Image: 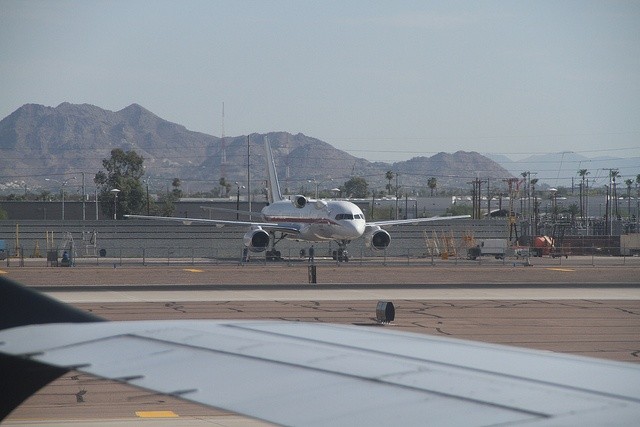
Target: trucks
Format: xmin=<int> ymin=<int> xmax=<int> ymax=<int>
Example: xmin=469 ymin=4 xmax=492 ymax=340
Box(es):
xmin=468 ymin=238 xmax=507 ymax=260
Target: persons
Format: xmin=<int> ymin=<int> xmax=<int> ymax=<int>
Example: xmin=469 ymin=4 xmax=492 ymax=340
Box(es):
xmin=62 ymin=251 xmax=70 ymax=263
xmin=535 ymin=233 xmax=546 ymax=257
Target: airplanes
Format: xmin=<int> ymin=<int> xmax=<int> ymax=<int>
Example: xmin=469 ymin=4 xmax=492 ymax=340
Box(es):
xmin=123 ymin=134 xmax=472 ymax=261
